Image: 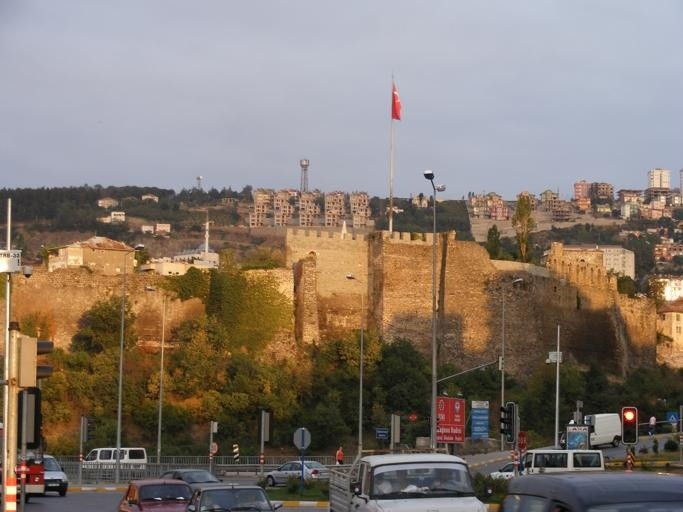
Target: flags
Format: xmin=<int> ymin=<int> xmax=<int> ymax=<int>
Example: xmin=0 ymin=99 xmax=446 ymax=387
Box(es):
xmin=390 ymin=80 xmax=402 ymax=123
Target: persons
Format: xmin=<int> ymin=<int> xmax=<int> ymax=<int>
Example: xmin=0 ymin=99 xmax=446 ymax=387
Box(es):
xmin=645 ymin=413 xmax=658 ymax=436
xmin=334 ymin=444 xmax=345 ymax=466
xmin=200 ymin=495 xmax=221 ymax=511
xmin=241 ymin=493 xmax=258 ymax=507
xmin=374 ymin=473 xmax=393 ymax=496
xmin=394 ymin=469 xmax=418 ymax=494
xmin=429 ymin=468 xmax=453 ymax=491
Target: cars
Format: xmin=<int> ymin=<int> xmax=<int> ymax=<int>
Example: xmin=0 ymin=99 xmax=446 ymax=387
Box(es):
xmin=40 ymin=455 xmax=70 ymax=496
xmin=264 ymin=459 xmax=330 ymax=487
xmin=118 ymin=466 xmax=285 ymax=511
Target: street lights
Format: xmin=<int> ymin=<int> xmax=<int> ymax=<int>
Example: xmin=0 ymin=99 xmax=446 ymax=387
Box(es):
xmin=146 ymin=282 xmax=162 ymax=463
xmin=423 ymin=167 xmax=443 ymax=450
xmin=345 ymin=272 xmax=365 ymax=450
xmin=497 ymin=278 xmax=523 ymax=446
xmin=115 ymin=242 xmax=145 ymax=482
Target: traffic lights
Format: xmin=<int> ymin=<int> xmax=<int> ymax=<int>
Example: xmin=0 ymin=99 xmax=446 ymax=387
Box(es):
xmin=498 ymin=405 xmax=515 ymax=435
xmin=19 ymin=336 xmax=54 ymax=387
xmin=623 ymin=411 xmax=635 ymax=442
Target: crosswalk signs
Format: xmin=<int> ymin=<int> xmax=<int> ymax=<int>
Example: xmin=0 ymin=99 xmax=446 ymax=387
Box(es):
xmin=667 ymin=411 xmax=679 ymax=422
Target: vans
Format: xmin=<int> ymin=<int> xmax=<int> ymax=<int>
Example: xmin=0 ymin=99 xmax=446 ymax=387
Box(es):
xmin=559 ymin=412 xmax=621 ymax=451
xmin=82 ymin=445 xmax=148 ymax=471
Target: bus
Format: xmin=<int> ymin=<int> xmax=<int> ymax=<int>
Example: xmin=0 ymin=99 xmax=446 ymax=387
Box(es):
xmin=0 ymin=410 xmax=47 ymax=506
